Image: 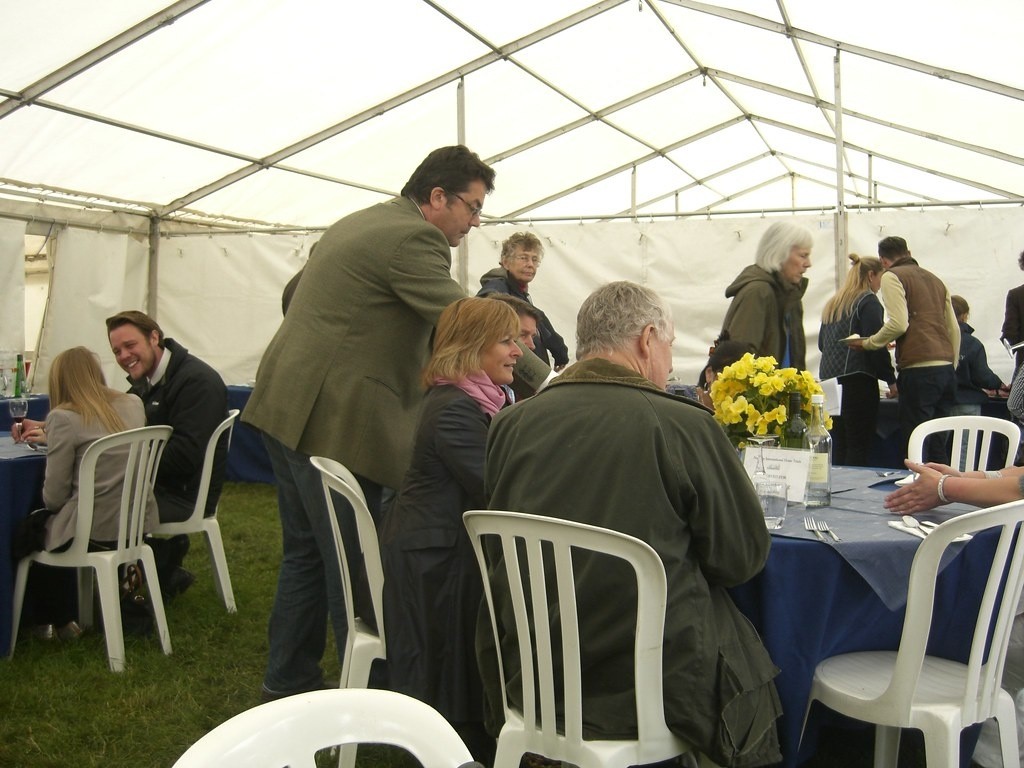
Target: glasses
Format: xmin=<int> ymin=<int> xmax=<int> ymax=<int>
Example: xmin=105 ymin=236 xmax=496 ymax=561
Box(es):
xmin=438 ymin=186 xmax=482 ymax=218
xmin=507 ymin=254 xmax=541 ymax=268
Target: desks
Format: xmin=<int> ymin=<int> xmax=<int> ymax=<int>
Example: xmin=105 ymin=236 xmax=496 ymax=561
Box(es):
xmin=0 ymin=431 xmax=47 ymax=656
xmin=749 ymin=463 xmax=1024 ymax=768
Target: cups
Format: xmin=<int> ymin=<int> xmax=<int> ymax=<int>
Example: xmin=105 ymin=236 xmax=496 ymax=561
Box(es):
xmin=755 ymin=483 xmax=787 ymax=530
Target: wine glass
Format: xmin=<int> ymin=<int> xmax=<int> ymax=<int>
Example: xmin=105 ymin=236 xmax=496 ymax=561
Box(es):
xmin=9 ymin=400 xmax=28 ymax=444
xmin=2 ymin=370 xmax=13 ymax=397
xmin=748 ymin=436 xmax=775 ymax=483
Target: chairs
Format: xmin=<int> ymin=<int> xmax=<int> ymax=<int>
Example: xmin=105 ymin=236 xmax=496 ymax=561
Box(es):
xmin=7 ymin=424 xmax=173 ymax=673
xmin=168 ymin=688 xmax=475 ymax=768
xmin=907 ymin=415 xmax=1020 ymax=477
xmin=463 ymin=511 xmax=691 ymax=768
xmin=310 ymin=455 xmax=385 ymax=768
xmin=792 ymin=500 xmax=1024 ymax=768
xmin=147 ymin=410 xmax=241 ymax=613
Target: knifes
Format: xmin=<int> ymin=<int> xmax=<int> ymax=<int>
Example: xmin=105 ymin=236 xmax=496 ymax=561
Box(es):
xmin=918 ymin=522 xmax=934 ymax=530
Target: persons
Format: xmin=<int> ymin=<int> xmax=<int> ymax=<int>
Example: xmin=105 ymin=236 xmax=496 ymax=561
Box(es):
xmin=238 ymin=145 xmax=559 ymax=698
xmin=11 ymin=311 xmax=229 ymax=644
xmin=488 ymin=281 xmax=784 ymax=768
xmin=883 ymin=459 xmax=1024 ymax=768
xmin=282 ymin=231 xmax=569 ymax=723
xmin=696 ymin=221 xmax=812 ymax=411
xmin=819 ymin=236 xmax=1024 ymax=471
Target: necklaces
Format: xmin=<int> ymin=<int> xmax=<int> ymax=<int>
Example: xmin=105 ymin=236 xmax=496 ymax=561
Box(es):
xmin=701 ymin=397 xmax=705 ymax=405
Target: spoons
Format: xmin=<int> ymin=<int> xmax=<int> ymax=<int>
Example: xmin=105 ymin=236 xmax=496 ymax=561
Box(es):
xmin=902 ymin=515 xmax=928 ymax=536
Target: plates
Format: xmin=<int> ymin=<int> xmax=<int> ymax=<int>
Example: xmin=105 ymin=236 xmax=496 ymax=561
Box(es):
xmin=839 ymin=337 xmax=868 ymax=343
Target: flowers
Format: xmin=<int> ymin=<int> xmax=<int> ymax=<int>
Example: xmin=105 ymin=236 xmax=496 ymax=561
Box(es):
xmin=709 ymin=352 xmax=834 ymax=447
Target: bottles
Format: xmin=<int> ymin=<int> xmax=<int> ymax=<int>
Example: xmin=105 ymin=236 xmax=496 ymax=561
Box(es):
xmin=803 ymin=394 xmax=832 ymax=506
xmin=779 ymin=393 xmax=807 ymax=450
xmin=15 ymin=354 xmax=27 ymax=398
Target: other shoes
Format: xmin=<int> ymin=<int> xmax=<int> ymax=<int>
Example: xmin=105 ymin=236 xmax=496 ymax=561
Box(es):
xmin=174 ymin=568 xmax=195 ymax=594
xmin=58 ymin=621 xmax=82 ymax=639
xmin=23 ymin=624 xmax=58 ymax=644
xmin=262 ymin=673 xmax=330 ymax=703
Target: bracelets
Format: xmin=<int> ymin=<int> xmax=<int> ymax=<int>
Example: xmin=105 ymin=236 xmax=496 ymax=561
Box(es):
xmin=983 ymin=471 xmax=1002 ymax=479
xmin=938 ymin=474 xmax=952 ymax=503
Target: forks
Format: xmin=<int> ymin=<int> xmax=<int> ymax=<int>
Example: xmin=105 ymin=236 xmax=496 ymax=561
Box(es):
xmin=804 ymin=517 xmax=824 ymax=541
xmin=817 ymin=521 xmax=840 ymax=541
xmin=876 ymin=472 xmax=894 ymax=476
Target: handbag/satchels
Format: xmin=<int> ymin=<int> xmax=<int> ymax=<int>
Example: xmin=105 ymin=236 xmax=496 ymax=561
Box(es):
xmin=119 ymin=564 xmax=153 ymax=635
